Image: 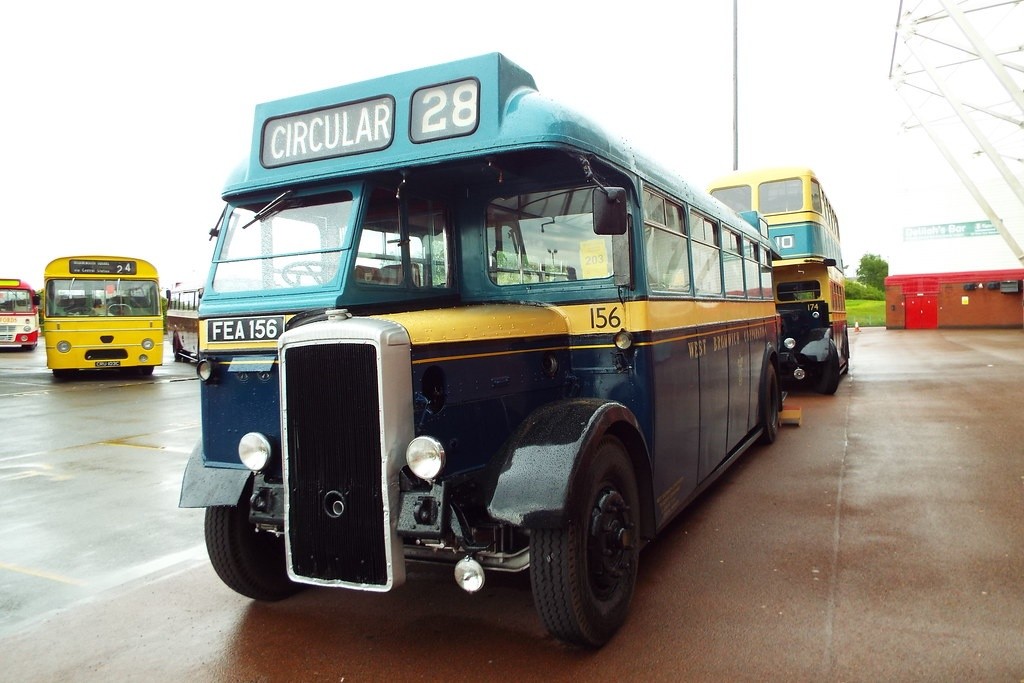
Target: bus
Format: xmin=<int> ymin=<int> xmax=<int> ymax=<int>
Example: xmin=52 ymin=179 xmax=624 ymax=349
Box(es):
xmin=32 ymin=255 xmax=163 ymax=376
xmin=704 ymin=164 xmax=849 ymax=396
xmin=166 ymin=287 xmax=203 ymax=363
xmin=0 ymin=279 xmax=39 ymax=350
xmin=178 ymin=52 xmax=780 ymax=654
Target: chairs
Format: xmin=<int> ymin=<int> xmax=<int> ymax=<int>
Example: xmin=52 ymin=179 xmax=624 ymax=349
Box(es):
xmin=374 ymin=262 xmax=421 ymax=288
